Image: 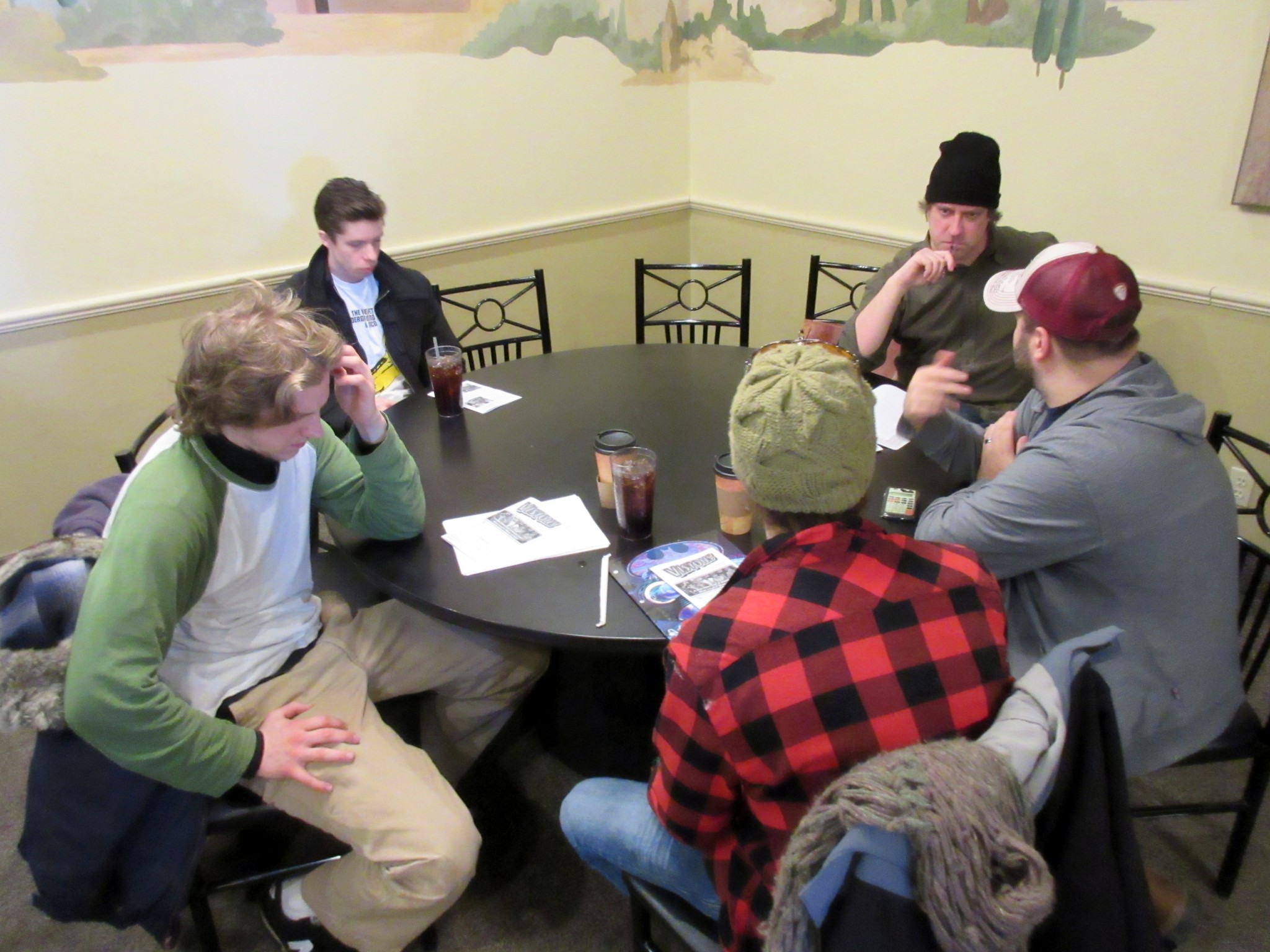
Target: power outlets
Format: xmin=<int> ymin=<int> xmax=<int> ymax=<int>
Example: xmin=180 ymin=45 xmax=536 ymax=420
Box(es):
xmin=1229 ymin=466 xmax=1255 ymax=508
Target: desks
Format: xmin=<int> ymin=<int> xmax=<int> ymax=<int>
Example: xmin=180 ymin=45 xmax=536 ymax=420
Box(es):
xmin=338 ymin=343 xmax=968 ymax=783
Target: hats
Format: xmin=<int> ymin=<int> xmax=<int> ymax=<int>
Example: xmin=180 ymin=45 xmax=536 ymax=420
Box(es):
xmin=924 ymin=131 xmax=1002 ymax=210
xmin=727 ymin=343 xmax=878 ymax=514
xmin=982 ymin=242 xmax=1143 ymax=344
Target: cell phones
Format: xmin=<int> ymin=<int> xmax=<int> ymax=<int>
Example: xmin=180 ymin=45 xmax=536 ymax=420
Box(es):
xmin=879 ymin=487 xmax=920 ymax=521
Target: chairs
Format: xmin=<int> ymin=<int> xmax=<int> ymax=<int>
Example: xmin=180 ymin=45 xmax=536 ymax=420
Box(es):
xmin=431 ymin=269 xmax=553 ymax=374
xmin=634 ymin=257 xmax=750 ymax=348
xmin=622 ymin=625 xmax=1177 ymax=952
xmin=1131 ymin=409 xmax=1270 ymax=899
xmin=804 ymin=254 xmax=882 ymax=325
xmin=0 ymin=469 xmax=422 ymax=952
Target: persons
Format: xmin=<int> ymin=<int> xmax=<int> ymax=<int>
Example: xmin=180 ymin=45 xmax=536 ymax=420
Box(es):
xmin=62 ymin=277 xmax=550 ymax=952
xmin=558 ymin=340 xmax=1016 ymax=952
xmin=896 ymin=236 xmax=1247 ymax=787
xmin=838 ymin=132 xmax=1059 ymax=429
xmin=271 ymin=176 xmax=467 ymax=440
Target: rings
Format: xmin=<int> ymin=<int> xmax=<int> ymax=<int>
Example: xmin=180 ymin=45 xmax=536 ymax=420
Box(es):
xmin=985 ymin=438 xmax=992 ymax=444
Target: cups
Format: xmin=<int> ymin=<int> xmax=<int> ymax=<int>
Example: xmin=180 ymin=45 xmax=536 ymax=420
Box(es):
xmin=425 ymin=346 xmax=464 ymax=418
xmin=610 ymin=447 xmax=657 ymax=541
xmin=713 ymin=452 xmax=753 ymax=535
xmin=594 ymin=429 xmax=637 ymax=483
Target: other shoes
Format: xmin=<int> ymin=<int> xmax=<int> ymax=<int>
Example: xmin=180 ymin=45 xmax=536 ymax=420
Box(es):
xmin=1144 ymin=865 xmax=1188 ymax=938
xmin=255 ymin=878 xmax=347 ymax=952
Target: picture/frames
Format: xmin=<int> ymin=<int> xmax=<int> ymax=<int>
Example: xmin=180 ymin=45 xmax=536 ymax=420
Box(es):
xmin=1231 ymin=33 xmax=1270 ymax=208
